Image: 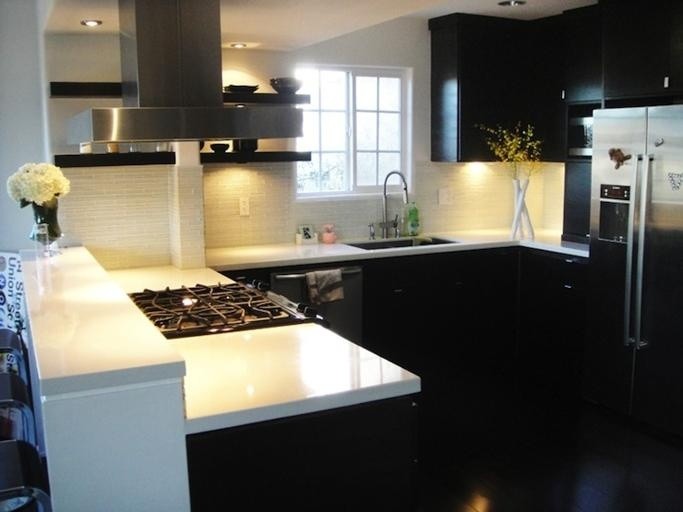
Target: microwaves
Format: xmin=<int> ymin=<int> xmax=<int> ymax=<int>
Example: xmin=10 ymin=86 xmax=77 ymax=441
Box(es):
xmin=566 ymin=114 xmax=601 ymax=157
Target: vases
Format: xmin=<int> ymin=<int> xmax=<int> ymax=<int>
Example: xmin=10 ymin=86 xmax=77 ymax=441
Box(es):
xmin=513 ymin=178 xmax=534 ymax=241
xmin=509 ymin=177 xmax=529 ymax=241
xmin=29 ymin=197 xmax=62 ymax=244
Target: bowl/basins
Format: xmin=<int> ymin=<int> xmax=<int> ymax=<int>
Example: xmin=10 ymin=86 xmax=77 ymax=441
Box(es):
xmin=198 ymin=139 xmax=204 ymax=152
xmin=210 ymin=144 xmax=229 ymax=153
xmin=232 ymin=138 xmax=258 ymax=151
xmin=269 ymin=73 xmax=301 ymax=94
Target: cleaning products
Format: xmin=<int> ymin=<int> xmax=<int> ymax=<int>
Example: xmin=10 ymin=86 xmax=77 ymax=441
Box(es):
xmin=405 ymin=202 xmax=422 ymax=236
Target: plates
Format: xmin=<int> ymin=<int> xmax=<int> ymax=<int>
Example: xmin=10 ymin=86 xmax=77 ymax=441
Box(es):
xmin=224 ymin=84 xmax=259 ymax=94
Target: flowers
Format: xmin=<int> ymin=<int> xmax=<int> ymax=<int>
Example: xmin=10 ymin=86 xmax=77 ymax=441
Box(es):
xmin=6 ymin=163 xmax=71 ymax=204
xmin=475 ymin=121 xmax=521 ymax=176
xmin=520 ymin=122 xmax=542 ymax=176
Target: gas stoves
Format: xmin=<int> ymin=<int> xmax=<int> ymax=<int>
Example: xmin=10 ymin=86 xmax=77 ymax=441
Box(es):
xmin=124 ymin=276 xmax=332 ymax=340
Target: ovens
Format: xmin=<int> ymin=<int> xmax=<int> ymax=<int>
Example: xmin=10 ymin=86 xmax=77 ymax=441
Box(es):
xmin=268 ymin=267 xmax=365 ymax=346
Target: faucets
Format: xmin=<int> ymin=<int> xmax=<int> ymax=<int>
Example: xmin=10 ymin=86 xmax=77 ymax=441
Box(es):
xmin=380 ymin=170 xmax=409 ymax=238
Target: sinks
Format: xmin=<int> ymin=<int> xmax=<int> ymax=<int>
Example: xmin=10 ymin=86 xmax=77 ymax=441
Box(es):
xmin=343 ymin=237 xmax=459 ymax=250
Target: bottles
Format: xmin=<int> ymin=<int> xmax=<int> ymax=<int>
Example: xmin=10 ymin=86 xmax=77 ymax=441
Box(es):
xmin=408 ymin=200 xmax=420 ymax=236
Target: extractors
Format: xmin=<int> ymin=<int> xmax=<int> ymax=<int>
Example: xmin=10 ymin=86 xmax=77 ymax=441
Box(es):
xmin=64 ymin=0 xmax=311 ymax=144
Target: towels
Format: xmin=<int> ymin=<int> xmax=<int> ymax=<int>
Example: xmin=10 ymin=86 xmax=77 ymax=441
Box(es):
xmin=306 ymin=268 xmax=344 ymax=305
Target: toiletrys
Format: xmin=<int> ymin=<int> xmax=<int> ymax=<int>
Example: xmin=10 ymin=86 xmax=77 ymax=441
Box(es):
xmin=322 ymin=224 xmax=339 ymax=244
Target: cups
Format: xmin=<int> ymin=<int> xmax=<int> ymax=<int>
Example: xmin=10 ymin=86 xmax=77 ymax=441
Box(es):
xmin=75 ymin=139 xmax=175 ymax=154
xmin=321 ymin=232 xmax=338 ymax=244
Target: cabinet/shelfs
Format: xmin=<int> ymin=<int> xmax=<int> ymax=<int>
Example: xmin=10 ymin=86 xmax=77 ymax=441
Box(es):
xmin=460 ymin=15 xmax=568 ymax=160
xmin=605 ymin=2 xmax=683 ymax=108
xmin=520 ymin=248 xmax=625 ymax=412
xmin=564 ymin=4 xmax=602 ymax=163
xmin=365 ymin=245 xmax=521 ymax=415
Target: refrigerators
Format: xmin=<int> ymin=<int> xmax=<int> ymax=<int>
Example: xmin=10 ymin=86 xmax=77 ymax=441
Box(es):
xmin=583 ymin=106 xmax=680 ymax=442
